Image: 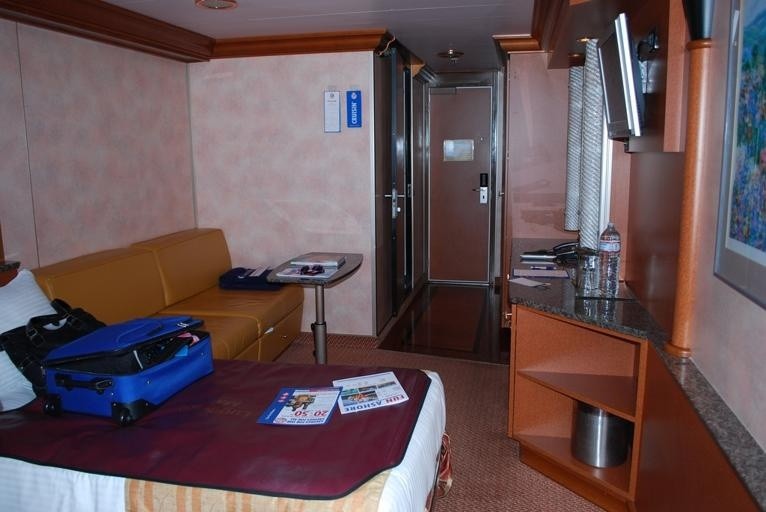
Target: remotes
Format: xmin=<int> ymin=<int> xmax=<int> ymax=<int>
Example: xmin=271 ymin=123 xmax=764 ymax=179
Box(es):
xmin=520 ymin=253 xmax=558 ymax=260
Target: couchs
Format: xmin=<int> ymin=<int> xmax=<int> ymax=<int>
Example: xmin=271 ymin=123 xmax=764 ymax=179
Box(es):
xmin=29 ymin=228 xmax=304 ymax=363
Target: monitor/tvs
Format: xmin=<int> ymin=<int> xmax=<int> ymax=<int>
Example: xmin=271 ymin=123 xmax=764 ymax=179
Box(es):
xmin=597 ymin=12 xmax=646 ymax=141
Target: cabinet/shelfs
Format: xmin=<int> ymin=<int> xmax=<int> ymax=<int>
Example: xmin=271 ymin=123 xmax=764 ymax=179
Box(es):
xmin=499 ymin=237 xmax=647 ymax=511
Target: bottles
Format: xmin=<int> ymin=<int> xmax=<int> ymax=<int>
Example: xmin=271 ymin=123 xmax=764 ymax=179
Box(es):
xmin=598 ymin=223 xmax=621 ymax=294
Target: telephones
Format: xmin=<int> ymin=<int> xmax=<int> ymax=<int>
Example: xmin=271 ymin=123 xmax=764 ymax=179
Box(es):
xmin=553 ymin=242 xmax=580 ymax=257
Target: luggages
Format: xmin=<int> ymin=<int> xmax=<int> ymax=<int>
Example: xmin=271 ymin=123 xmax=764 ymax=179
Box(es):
xmin=41 ymin=311 xmax=216 ymax=427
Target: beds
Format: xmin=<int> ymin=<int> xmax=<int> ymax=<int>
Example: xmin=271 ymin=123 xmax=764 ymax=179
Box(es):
xmin=0 ymin=266 xmax=452 ymax=512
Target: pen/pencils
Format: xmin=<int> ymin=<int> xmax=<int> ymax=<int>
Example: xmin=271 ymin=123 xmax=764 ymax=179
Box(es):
xmin=530 ymin=265 xmax=557 ymax=270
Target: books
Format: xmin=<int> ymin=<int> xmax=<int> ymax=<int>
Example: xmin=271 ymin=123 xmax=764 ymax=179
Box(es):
xmin=254 ymin=385 xmax=343 ymax=425
xmin=289 ymin=253 xmax=345 ymax=267
xmin=333 ymin=370 xmax=409 ymax=416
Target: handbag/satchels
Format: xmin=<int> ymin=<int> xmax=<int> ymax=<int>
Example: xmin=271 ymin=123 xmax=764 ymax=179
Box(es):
xmin=0 ymin=296 xmax=107 ymax=398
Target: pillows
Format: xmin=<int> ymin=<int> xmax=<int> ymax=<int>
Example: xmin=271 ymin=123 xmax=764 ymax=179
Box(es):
xmin=0 ymin=268 xmax=64 ymax=414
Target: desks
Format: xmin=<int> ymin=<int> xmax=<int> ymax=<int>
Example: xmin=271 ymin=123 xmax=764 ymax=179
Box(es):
xmin=266 ymin=251 xmax=363 ymax=366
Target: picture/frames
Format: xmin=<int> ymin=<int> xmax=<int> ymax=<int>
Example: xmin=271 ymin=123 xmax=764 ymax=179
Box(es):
xmin=711 ymin=1 xmax=766 ymax=314
